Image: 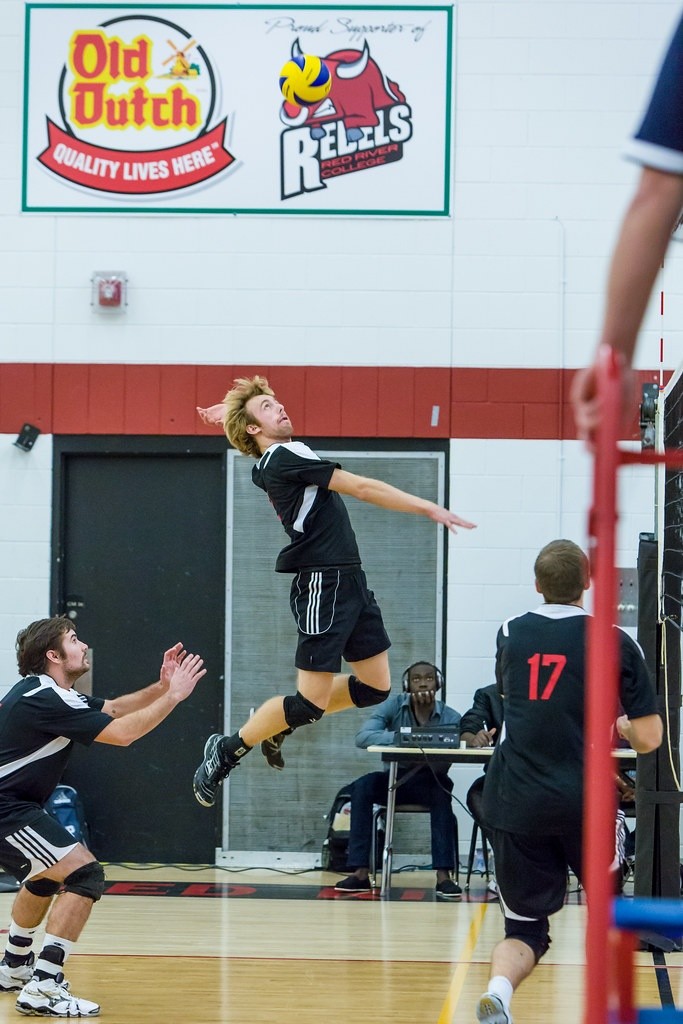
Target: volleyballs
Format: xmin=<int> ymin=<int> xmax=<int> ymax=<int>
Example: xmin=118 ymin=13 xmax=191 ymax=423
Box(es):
xmin=280 ymin=54 xmax=332 ymax=107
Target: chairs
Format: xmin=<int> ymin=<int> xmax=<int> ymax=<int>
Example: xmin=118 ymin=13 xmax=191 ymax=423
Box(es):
xmin=371 ymin=805 xmax=460 ymax=888
xmin=465 ymin=820 xmax=491 ymax=892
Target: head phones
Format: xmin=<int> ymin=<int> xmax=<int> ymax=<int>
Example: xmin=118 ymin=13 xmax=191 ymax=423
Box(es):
xmin=402 ymin=664 xmax=445 ymax=694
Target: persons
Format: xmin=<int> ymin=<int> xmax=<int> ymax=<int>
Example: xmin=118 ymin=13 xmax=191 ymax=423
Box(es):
xmin=570 ymin=0 xmax=683 ymax=454
xmin=334 ymin=660 xmax=462 ymax=896
xmin=193 ymin=376 xmax=477 ymax=807
xmin=0 ymin=617 xmax=207 ymax=1018
xmin=479 ymin=540 xmax=663 ymax=1024
xmin=459 ymin=683 xmax=504 ymax=829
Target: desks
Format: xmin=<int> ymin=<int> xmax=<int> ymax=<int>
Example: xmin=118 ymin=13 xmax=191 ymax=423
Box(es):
xmin=366 ymin=746 xmax=638 ymax=898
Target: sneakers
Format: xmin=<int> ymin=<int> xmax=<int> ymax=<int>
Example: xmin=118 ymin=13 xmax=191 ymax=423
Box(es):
xmin=249 ymin=708 xmax=287 ymax=771
xmin=190 ymin=730 xmax=242 ymax=810
xmin=488 ymin=877 xmax=499 ymax=897
xmin=476 ymin=991 xmax=512 ymax=1024
xmin=13 ymin=980 xmax=102 ymax=1017
xmin=0 ymin=957 xmax=38 ymax=991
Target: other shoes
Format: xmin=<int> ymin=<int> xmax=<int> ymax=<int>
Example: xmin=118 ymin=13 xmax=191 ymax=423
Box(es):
xmin=334 ymin=874 xmax=372 ymax=894
xmin=435 ymin=877 xmax=463 ymax=898
